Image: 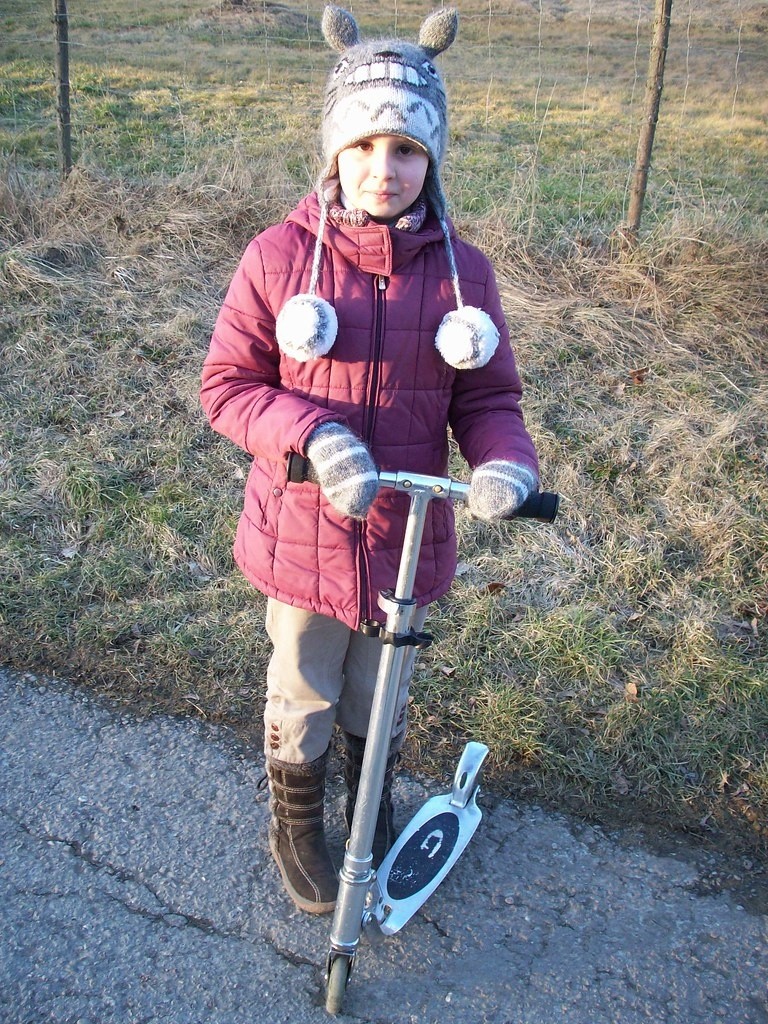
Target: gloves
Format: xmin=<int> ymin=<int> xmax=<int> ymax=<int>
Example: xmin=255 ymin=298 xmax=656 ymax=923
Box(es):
xmin=304 ymin=420 xmax=378 ymax=520
xmin=463 ymin=458 xmax=539 ymax=522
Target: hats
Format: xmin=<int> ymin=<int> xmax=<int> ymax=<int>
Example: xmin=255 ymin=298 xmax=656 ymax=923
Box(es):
xmin=316 ymin=5 xmax=458 ymax=223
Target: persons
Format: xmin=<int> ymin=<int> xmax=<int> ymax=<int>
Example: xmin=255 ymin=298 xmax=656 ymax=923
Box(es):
xmin=198 ymin=5 xmax=540 ymax=913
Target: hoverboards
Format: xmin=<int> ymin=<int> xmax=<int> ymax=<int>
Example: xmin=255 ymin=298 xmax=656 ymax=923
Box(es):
xmin=283 ymin=447 xmax=560 ymax=1012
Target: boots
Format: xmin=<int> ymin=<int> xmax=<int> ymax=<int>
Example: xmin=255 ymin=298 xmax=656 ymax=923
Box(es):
xmin=340 ymin=729 xmax=406 ymax=873
xmin=262 ymin=754 xmax=340 ymax=913
